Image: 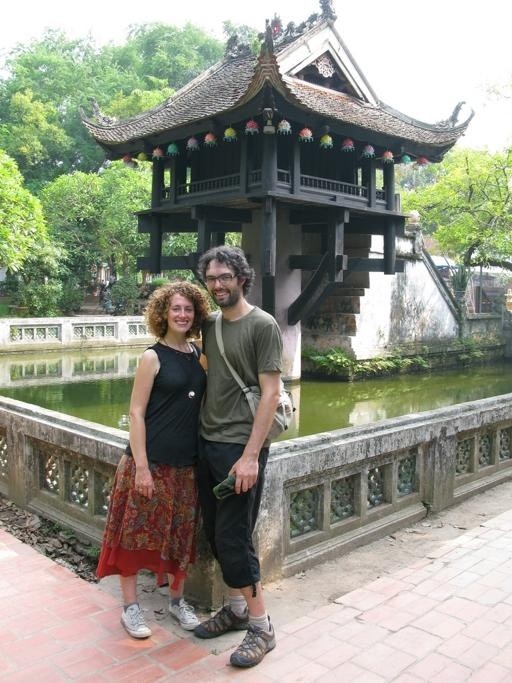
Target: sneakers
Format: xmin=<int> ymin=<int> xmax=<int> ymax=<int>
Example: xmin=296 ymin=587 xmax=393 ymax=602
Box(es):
xmin=194 ymin=604 xmax=248 ymax=643
xmin=121 ymin=603 xmax=152 ymax=639
xmin=230 ymin=615 xmax=275 ymax=667
xmin=168 ymin=598 xmax=202 ymax=630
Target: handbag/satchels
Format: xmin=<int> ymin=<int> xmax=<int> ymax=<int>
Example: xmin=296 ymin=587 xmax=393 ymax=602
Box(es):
xmin=245 ymin=380 xmax=294 ymax=439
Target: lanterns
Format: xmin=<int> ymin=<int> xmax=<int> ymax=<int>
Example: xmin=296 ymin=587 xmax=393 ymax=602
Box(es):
xmin=122 ymin=118 xmax=259 ymax=168
xmin=279 ymin=118 xmax=430 ymax=170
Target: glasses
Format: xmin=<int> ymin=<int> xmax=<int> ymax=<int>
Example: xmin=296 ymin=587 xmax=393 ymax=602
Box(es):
xmin=204 ymin=273 xmax=239 ymax=285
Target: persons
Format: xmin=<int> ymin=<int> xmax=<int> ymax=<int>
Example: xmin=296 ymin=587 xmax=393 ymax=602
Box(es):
xmin=93 ymin=282 xmax=210 ymax=640
xmin=190 ymin=245 xmax=284 ymax=669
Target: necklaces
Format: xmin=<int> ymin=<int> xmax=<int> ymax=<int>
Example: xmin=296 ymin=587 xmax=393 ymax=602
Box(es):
xmin=163 ymin=338 xmax=191 ymax=353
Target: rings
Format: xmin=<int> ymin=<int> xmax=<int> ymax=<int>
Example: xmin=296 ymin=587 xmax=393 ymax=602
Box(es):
xmin=138 ymin=489 xmax=143 ymax=492
